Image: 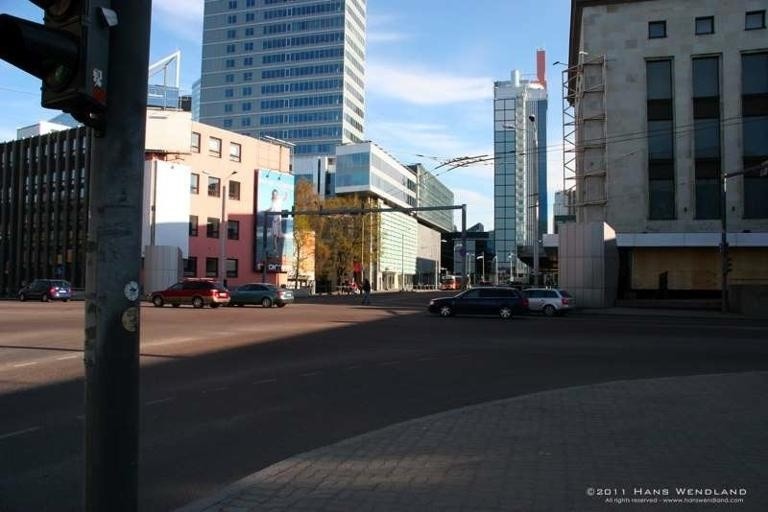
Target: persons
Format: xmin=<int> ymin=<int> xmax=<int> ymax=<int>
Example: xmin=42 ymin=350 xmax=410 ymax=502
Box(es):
xmin=363 ymin=279 xmax=374 ymax=309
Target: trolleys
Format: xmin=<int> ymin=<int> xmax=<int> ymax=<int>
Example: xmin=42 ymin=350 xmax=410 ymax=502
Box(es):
xmin=349 ymin=288 xmax=364 ymax=304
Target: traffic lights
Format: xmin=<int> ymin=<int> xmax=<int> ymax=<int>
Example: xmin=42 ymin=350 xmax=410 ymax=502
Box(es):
xmin=0 ymin=0 xmax=119 ymax=138
xmin=721 ymin=254 xmax=734 ymax=276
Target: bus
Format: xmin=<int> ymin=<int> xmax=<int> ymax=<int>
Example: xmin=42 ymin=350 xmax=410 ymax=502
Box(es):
xmin=440 ymin=274 xmax=465 ymax=290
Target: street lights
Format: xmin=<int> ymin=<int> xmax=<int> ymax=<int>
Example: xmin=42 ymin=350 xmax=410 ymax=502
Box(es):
xmin=453 ymin=243 xmax=519 ymax=288
xmin=402 ymin=227 xmax=414 ymax=293
xmin=435 ymin=239 xmax=448 ymax=290
xmin=498 ymin=114 xmax=539 ymax=291
xmin=218 ymin=170 xmax=240 ymax=285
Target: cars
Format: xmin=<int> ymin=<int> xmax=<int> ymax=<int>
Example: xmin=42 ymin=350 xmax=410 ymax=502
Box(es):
xmin=223 ymin=283 xmax=297 ymax=310
xmin=150 ymin=276 xmax=234 ymax=311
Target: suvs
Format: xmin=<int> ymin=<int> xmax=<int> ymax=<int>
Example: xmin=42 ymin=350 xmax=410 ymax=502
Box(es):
xmin=426 ymin=285 xmax=527 ymax=320
xmin=518 ymin=287 xmax=577 ymax=317
xmin=16 ymin=277 xmax=73 ymax=303
